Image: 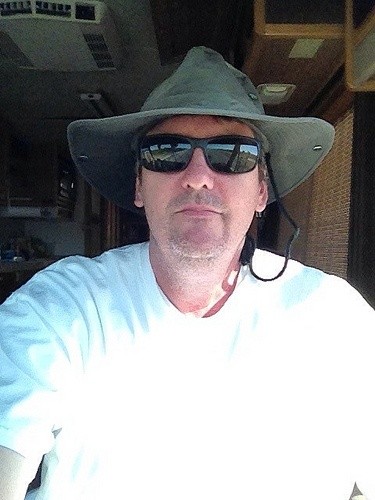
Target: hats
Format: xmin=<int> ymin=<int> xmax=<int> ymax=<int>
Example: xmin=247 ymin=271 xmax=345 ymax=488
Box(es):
xmin=67 ymin=46 xmax=337 ymax=216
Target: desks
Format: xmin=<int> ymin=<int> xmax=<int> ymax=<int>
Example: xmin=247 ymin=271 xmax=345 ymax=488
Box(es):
xmin=0 ymin=255 xmax=58 ymax=282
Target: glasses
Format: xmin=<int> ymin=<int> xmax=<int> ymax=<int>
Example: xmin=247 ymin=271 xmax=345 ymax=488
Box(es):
xmin=135 ymin=133 xmax=266 ymax=175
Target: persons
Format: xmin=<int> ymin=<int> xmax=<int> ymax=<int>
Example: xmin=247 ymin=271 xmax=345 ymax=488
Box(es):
xmin=0 ymin=46 xmax=375 ymax=500
